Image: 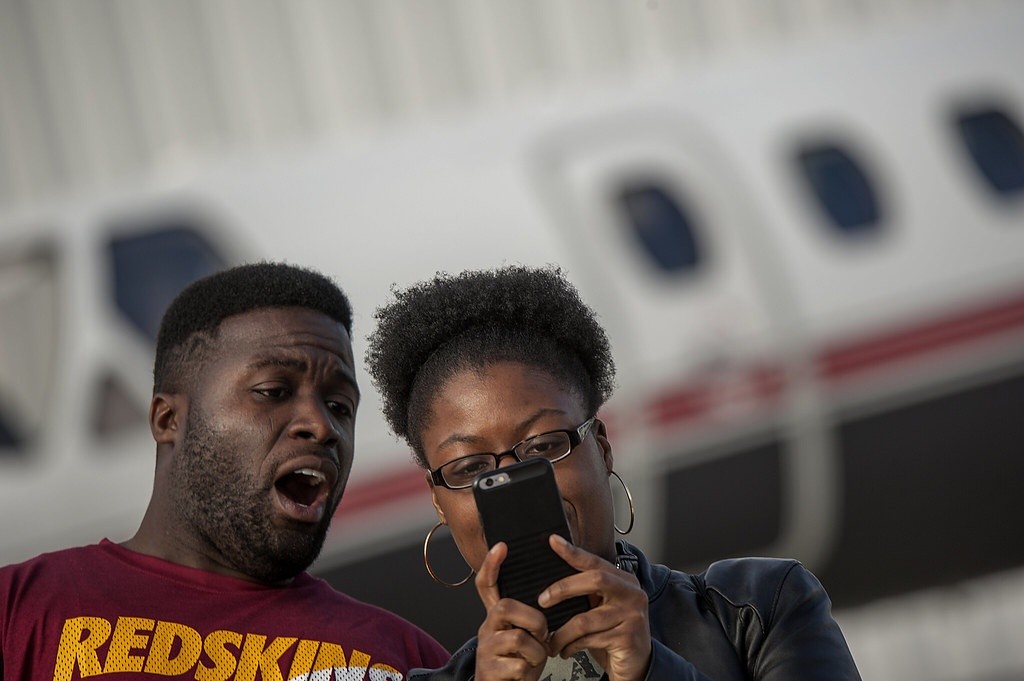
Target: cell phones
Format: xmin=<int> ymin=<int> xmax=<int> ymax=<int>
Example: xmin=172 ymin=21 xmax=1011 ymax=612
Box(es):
xmin=473 ymin=457 xmax=591 ymax=636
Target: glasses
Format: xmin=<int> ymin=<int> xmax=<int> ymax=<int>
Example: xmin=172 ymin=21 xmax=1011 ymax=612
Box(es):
xmin=423 ymin=415 xmax=597 ymax=490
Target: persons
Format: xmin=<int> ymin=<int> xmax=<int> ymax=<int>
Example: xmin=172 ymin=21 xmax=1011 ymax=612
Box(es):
xmin=370 ymin=267 xmax=864 ymax=681
xmin=0 ymin=262 xmax=452 ymax=681
